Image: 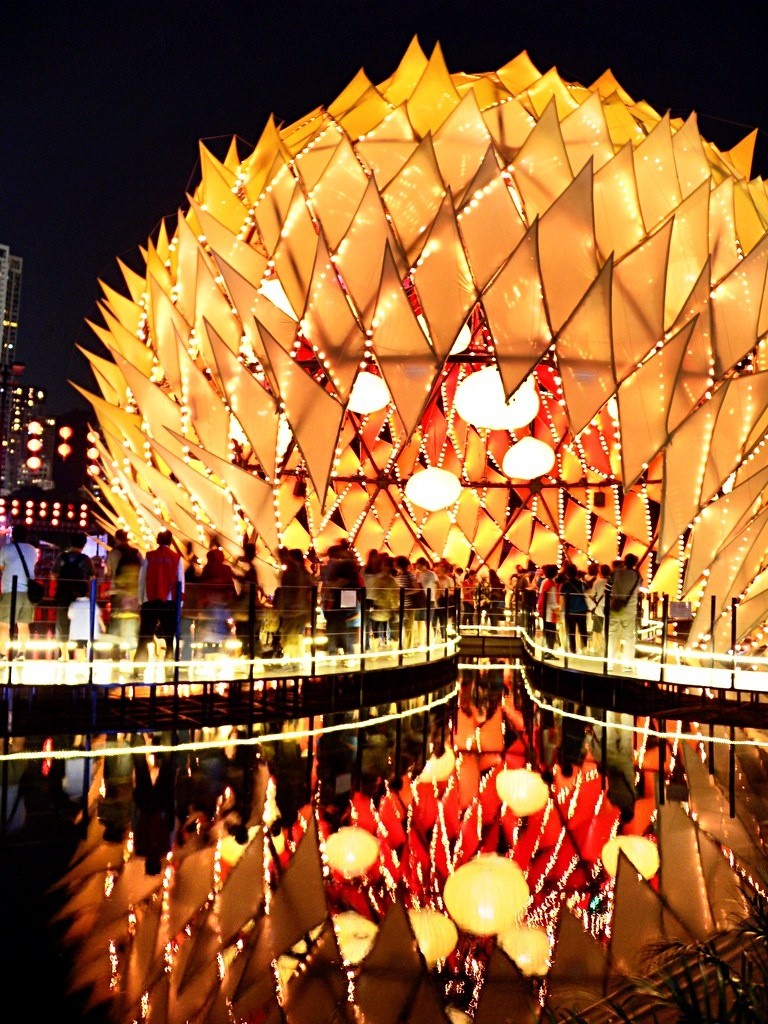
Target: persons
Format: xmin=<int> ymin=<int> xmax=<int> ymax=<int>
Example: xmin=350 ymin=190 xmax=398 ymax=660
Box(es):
xmin=17 ymin=730 xmax=323 ymax=876
xmin=520 ymin=688 xmax=689 ymax=820
xmin=0 ymin=524 xmax=625 ymax=660
xmin=317 ymin=691 xmax=463 ymax=826
xmin=462 ymin=657 xmax=511 ymax=727
xmin=603 ymin=553 xmax=643 ymax=671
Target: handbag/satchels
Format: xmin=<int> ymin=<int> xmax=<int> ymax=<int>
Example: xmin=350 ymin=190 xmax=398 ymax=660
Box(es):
xmin=610 ymin=596 xmax=625 ymax=612
xmin=592 ymin=614 xmax=604 ymax=632
xmin=407 ymin=571 xmax=428 ymax=609
xmin=27 ymin=578 xmax=46 ymax=604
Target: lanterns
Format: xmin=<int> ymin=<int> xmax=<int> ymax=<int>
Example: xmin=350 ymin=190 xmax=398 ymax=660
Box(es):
xmin=0 ymin=419 xmax=99 ymax=537
